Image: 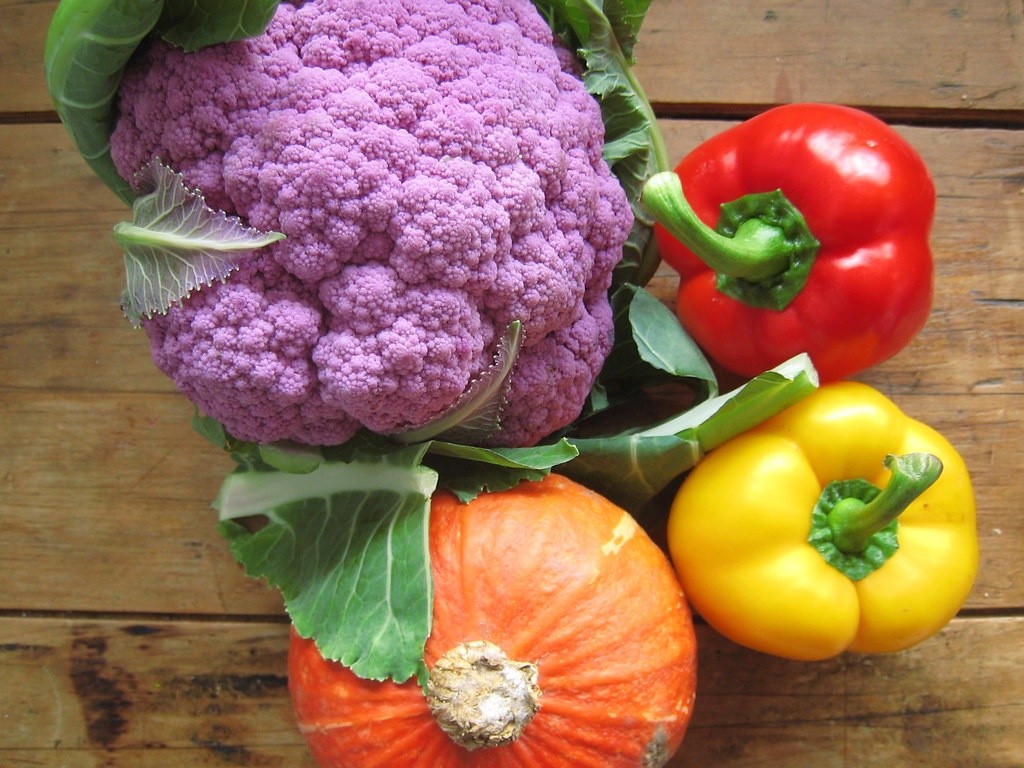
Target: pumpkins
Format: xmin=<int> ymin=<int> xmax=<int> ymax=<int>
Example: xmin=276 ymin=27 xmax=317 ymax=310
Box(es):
xmin=286 ymin=475 xmax=698 ymax=767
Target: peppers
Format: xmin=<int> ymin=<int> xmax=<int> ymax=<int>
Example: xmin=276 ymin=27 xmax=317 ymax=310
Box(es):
xmin=665 ymin=381 xmax=978 ymax=661
xmin=639 ymin=100 xmax=935 ymax=387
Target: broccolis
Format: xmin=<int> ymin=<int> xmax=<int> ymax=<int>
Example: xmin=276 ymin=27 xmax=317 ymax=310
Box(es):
xmin=44 ymin=0 xmax=820 ymax=682
